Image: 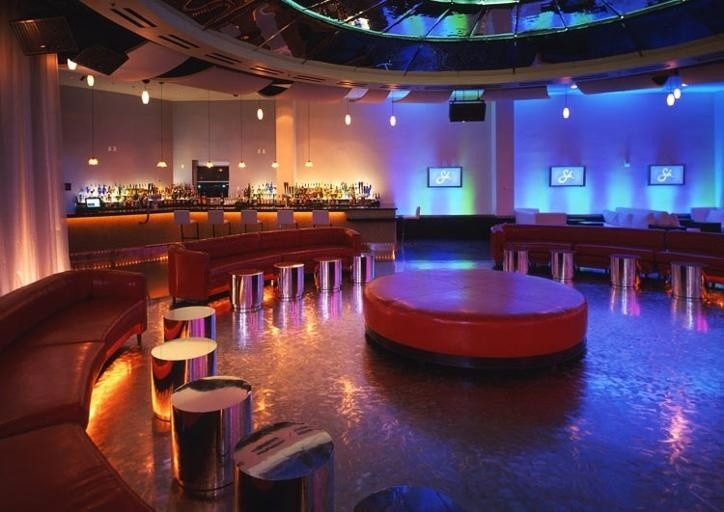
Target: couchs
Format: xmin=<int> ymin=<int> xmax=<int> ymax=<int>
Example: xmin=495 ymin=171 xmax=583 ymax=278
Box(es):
xmin=0 ymin=422 xmax=155 ymax=512
xmin=1 ymin=269 xmax=146 ymax=438
xmin=491 ymin=202 xmax=724 ymax=287
xmin=169 ymin=226 xmax=361 ymax=307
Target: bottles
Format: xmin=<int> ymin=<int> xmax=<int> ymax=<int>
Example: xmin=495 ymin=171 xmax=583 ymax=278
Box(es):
xmin=234 ymin=180 xmax=375 ymax=207
xmin=78 ymin=183 xmax=207 ymax=210
xmin=220 ymin=192 xmax=225 ymax=205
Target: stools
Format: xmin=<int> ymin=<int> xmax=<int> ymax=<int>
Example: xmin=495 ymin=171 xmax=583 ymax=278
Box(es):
xmin=503 ymin=247 xmax=702 ymax=300
xmin=363 ymin=270 xmax=588 ymax=378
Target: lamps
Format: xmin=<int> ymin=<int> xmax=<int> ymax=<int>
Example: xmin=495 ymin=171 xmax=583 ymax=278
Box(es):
xmin=86 ymin=75 xmax=94 ymax=87
xmin=89 ymin=90 xmax=98 ymax=165
xmin=345 ymin=100 xmax=351 ymax=126
xmin=257 ymin=94 xmax=264 ymax=120
xmin=563 ymin=85 xmax=570 ymax=119
xmin=667 ymin=77 xmax=675 ymax=107
xmin=157 ymin=81 xmax=168 ymax=169
xmin=272 ymin=99 xmax=279 ymax=168
xmin=205 ymin=89 xmax=213 ymax=168
xmin=674 ymin=75 xmax=681 ymax=100
xmin=390 ymin=102 xmax=396 ymax=126
xmin=304 ymin=101 xmax=313 ymax=166
xmin=141 ymin=79 xmax=150 ymax=104
xmin=238 ymin=95 xmax=246 ymax=169
xmin=67 ymin=59 xmax=78 ymax=72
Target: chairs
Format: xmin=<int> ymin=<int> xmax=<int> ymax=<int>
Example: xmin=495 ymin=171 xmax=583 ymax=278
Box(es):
xmin=312 ymin=210 xmax=332 ymax=228
xmin=277 ymin=210 xmax=298 ymax=229
xmin=208 ymin=209 xmax=231 ymax=237
xmin=400 ymin=206 xmax=420 ymax=247
xmin=241 ymin=210 xmax=263 ymax=232
xmin=174 ymin=210 xmax=198 ymax=241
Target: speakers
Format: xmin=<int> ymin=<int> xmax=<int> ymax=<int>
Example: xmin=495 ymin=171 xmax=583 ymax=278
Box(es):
xmin=8 ymin=14 xmax=80 ymax=56
xmin=70 ymin=39 xmax=129 ymax=76
xmin=449 ymin=99 xmax=487 ymax=123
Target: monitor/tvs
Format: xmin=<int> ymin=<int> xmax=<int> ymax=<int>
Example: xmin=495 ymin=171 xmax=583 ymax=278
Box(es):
xmin=549 ymin=166 xmax=586 ymax=187
xmin=647 ymin=163 xmax=685 ymax=186
xmin=427 ymin=166 xmax=462 ymax=188
